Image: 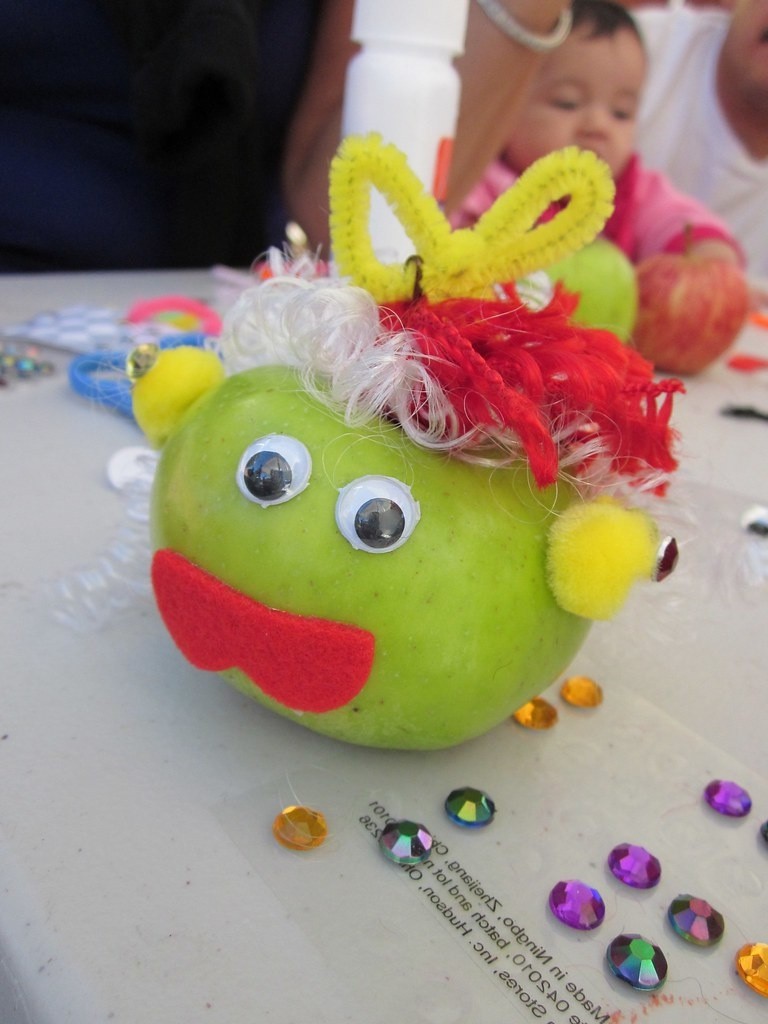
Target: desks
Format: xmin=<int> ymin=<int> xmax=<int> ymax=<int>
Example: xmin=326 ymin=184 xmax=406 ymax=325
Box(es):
xmin=0 ymin=269 xmax=768 ymax=1024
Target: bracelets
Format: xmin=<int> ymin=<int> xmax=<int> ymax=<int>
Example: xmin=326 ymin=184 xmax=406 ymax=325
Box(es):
xmin=478 ymin=0 xmax=576 ymax=53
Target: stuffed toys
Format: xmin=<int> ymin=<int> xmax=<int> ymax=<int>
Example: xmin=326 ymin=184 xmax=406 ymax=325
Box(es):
xmin=120 ymin=134 xmax=692 ymax=753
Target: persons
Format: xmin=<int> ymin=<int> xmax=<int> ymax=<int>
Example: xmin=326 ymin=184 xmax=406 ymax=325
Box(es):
xmin=441 ymin=2 xmax=766 ymax=286
xmin=448 ymin=6 xmax=753 ymax=284
xmin=0 ymin=0 xmax=358 ymax=270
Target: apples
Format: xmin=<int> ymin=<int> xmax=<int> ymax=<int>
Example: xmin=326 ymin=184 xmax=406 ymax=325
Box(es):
xmin=534 ymin=222 xmax=751 ymax=377
xmin=150 ymin=359 xmax=594 ymax=750
